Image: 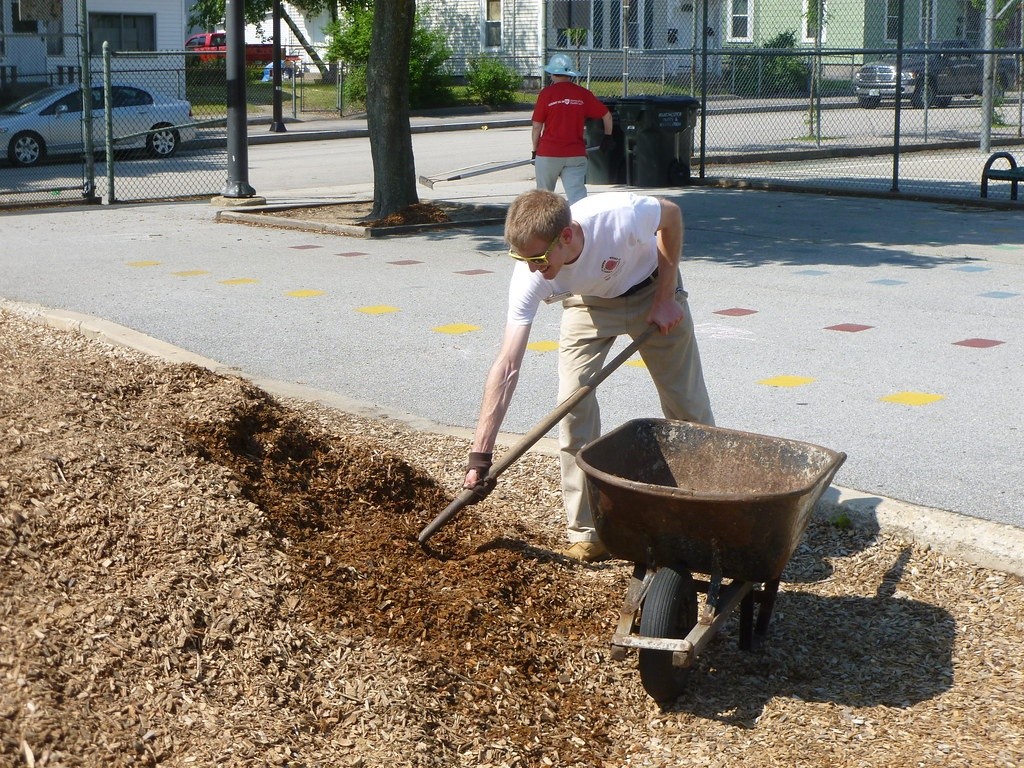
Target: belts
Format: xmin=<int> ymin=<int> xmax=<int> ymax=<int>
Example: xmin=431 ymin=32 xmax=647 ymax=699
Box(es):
xmin=617 ymin=266 xmax=659 ymax=298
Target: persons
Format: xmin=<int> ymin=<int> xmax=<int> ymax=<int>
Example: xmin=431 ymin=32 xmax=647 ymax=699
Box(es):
xmin=532 ymin=54 xmax=612 ymax=205
xmin=463 ymin=190 xmax=716 ymax=561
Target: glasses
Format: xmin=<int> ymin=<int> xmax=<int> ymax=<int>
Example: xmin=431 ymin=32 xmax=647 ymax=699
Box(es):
xmin=508 ymin=234 xmax=561 ymax=266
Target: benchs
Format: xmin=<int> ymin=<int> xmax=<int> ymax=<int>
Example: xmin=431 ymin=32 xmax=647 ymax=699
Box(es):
xmin=980 ymin=152 xmax=1024 ymax=200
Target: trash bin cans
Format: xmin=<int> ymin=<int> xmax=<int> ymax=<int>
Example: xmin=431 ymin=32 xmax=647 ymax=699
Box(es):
xmin=584 ymin=96 xmax=627 ymax=185
xmin=615 ymin=94 xmax=702 ymax=188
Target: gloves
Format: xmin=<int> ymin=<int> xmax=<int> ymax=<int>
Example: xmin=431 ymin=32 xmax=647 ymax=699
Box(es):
xmin=531 ymin=151 xmax=536 ymax=165
xmin=599 ymin=135 xmax=616 ymax=152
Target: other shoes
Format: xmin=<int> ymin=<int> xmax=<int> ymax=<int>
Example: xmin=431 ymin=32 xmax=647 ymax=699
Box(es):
xmin=566 ymin=541 xmax=609 ymax=563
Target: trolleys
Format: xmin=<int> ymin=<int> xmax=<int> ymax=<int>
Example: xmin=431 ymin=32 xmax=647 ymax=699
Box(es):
xmin=576 ymin=419 xmax=849 ymax=701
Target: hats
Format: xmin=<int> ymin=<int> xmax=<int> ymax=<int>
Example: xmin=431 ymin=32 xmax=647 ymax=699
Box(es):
xmin=544 ymin=54 xmax=581 ymax=76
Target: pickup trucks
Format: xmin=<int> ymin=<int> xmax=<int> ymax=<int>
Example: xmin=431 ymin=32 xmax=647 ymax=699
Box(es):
xmin=186 ymin=34 xmax=274 ymax=71
xmin=852 ymin=38 xmax=1019 ymax=109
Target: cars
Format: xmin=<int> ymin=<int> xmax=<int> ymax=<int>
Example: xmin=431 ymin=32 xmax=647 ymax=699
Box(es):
xmin=0 ymin=82 xmax=195 ymax=169
